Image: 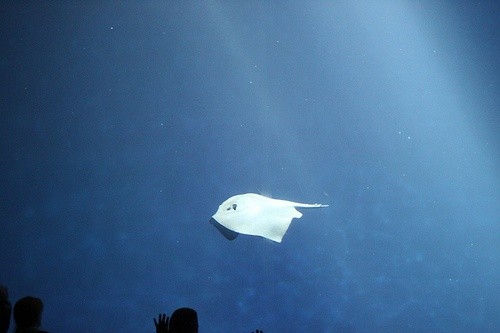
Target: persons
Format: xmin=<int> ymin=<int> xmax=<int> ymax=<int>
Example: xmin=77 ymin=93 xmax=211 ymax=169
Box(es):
xmin=153 ymin=307 xmax=267 ymax=333
xmin=0 ymin=284 xmax=49 ymax=333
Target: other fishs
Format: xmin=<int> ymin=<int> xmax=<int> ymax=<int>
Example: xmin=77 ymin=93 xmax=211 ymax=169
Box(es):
xmin=209 ymin=193 xmax=330 ymax=243
